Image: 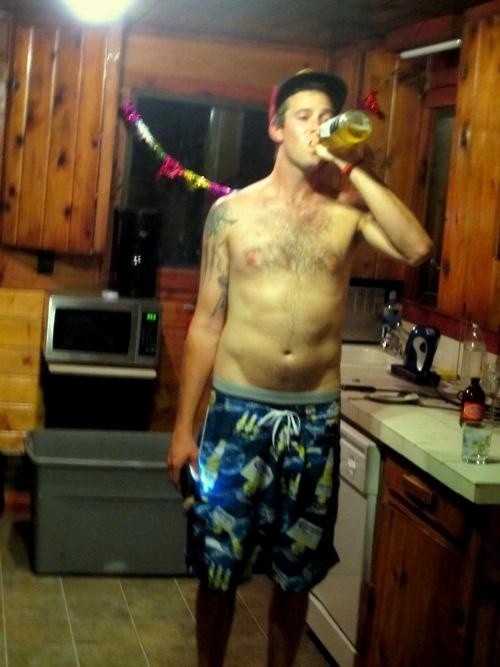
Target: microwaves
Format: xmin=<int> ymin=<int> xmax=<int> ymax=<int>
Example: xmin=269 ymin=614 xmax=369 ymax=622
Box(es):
xmin=41 ymin=288 xmax=162 ymax=367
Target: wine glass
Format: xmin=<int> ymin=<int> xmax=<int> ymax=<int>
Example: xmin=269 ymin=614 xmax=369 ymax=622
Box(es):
xmin=480 ymin=365 xmax=500 ymax=433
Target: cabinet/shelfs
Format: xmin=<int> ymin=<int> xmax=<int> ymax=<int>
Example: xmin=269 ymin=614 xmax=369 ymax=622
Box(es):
xmin=0 ymin=8 xmax=127 ymax=261
xmin=356 ymin=450 xmax=500 ymax=667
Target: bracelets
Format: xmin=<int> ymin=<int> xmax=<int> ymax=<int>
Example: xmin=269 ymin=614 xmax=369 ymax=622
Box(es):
xmin=343 ymin=156 xmax=361 ymax=177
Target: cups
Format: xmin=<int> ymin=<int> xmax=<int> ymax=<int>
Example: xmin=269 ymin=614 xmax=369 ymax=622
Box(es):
xmin=462 ymin=420 xmax=491 ymax=464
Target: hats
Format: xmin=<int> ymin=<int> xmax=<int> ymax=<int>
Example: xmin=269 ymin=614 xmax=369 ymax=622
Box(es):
xmin=272 ymin=67 xmax=346 ymax=117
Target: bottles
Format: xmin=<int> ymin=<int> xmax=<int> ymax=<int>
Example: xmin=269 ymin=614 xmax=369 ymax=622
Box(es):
xmin=383 ymin=291 xmax=403 ymax=354
xmin=318 ymin=111 xmax=372 ymax=149
xmin=456 ymin=320 xmax=485 ymax=428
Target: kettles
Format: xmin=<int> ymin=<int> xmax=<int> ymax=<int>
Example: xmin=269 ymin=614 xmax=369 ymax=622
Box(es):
xmin=405 ymin=325 xmax=441 ymax=376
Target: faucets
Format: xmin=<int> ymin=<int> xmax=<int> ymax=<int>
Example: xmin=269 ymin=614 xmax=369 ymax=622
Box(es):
xmin=381 ymin=331 xmax=405 ymax=358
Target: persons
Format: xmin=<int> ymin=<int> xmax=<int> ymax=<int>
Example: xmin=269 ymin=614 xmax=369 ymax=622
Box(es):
xmin=163 ymin=66 xmax=437 ymax=665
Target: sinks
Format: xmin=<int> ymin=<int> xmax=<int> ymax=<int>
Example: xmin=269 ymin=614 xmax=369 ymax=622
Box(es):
xmin=340 ymin=344 xmax=405 ymax=367
xmin=338 ymin=363 xmax=405 ymax=395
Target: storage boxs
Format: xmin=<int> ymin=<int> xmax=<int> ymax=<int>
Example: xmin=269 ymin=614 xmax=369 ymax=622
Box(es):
xmin=22 ymin=427 xmax=196 ymax=576
